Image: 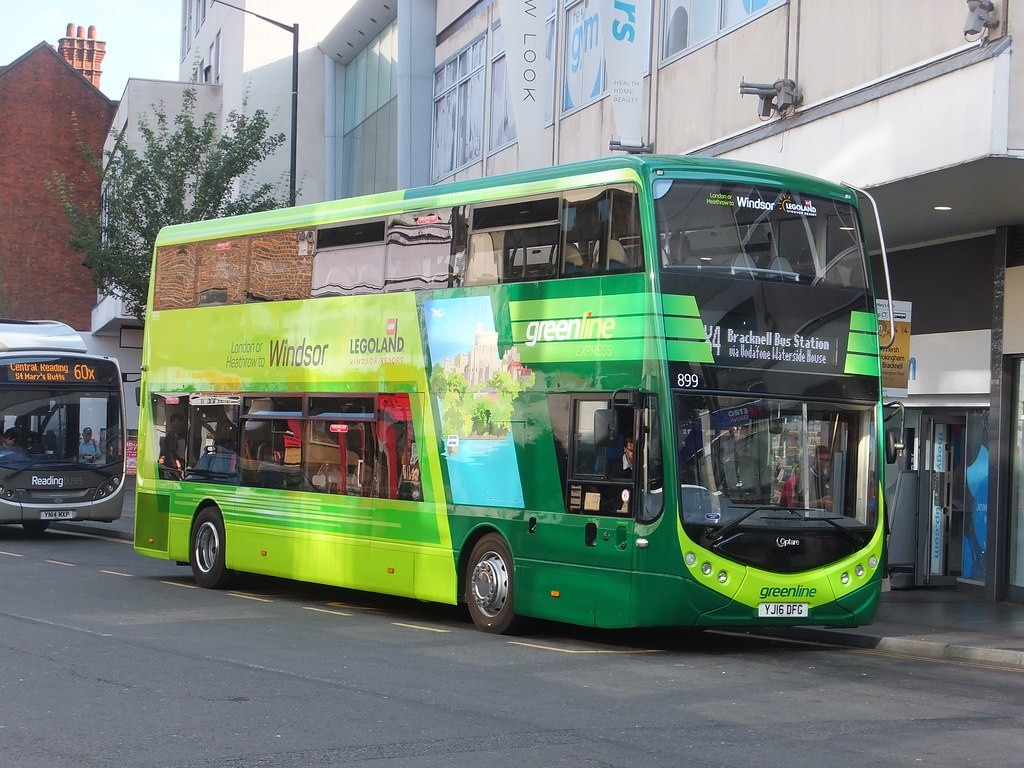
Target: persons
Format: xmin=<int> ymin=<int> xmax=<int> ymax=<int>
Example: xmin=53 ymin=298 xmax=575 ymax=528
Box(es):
xmin=607 ymin=436 xmax=633 ymax=478
xmin=157 ymin=411 xmax=189 ymax=478
xmin=0 ymin=414 xmax=29 ymax=447
xmin=79 ymin=427 xmax=101 ymax=459
xmin=779 ymin=445 xmax=833 ymax=511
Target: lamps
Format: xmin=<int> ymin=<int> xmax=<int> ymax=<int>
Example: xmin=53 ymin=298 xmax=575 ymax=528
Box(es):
xmin=772 ymin=79 xmax=803 ymax=113
xmin=963 ymin=0 xmax=999 ymax=42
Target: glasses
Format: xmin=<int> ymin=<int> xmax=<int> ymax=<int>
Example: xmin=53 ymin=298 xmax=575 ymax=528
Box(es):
xmin=627 ymin=448 xmax=633 ymax=452
xmin=8 ymin=438 xmax=13 ymax=440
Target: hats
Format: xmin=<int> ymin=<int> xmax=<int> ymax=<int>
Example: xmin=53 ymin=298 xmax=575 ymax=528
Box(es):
xmin=5 ymin=429 xmax=18 ymax=438
xmin=84 ymin=428 xmax=92 ymax=434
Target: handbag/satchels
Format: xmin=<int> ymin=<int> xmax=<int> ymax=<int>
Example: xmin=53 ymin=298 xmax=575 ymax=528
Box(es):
xmin=782 ymin=476 xmax=800 ymax=506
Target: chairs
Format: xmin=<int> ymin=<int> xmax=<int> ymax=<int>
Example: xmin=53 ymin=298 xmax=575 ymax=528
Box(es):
xmin=191 ymin=444 xmax=279 ymax=487
xmin=550 ymin=236 xmax=799 ymax=282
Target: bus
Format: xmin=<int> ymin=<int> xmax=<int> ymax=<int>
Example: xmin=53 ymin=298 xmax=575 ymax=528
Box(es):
xmin=130 ymin=153 xmax=898 ymax=635
xmin=0 ymin=318 xmax=128 ymax=535
